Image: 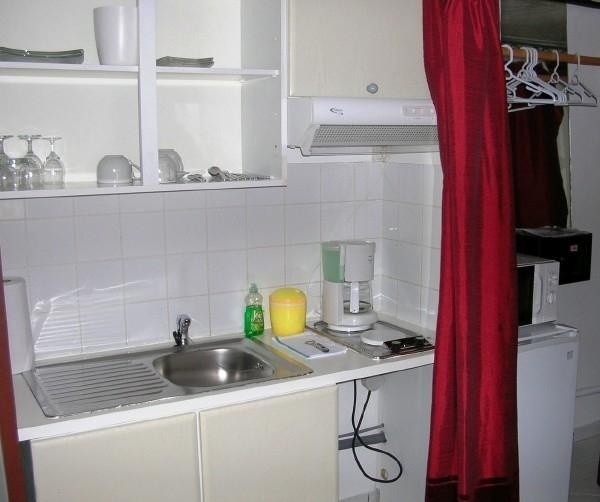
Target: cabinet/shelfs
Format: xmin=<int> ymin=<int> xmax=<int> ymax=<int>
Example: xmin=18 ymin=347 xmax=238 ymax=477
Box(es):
xmin=287 ymin=0 xmax=436 ymax=98
xmin=0 ymin=0 xmax=287 ymax=199
xmin=19 ymin=384 xmax=339 ymax=502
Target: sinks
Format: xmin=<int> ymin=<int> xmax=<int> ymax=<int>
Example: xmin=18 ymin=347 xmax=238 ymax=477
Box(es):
xmin=153 ymin=337 xmax=314 ymax=399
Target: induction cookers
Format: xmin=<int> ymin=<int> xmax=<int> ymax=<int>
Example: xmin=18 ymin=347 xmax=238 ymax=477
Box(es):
xmin=305 ymin=322 xmax=433 ymax=360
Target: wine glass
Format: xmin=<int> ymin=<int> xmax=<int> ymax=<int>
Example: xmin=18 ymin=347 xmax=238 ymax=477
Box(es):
xmin=0 ymin=133 xmax=65 ymax=192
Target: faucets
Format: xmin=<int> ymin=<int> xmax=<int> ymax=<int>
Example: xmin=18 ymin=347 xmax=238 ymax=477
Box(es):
xmin=176 ymin=314 xmax=191 ymax=344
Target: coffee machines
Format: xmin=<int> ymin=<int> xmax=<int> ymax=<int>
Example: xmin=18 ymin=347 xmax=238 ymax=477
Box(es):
xmin=320 ymin=239 xmax=377 ymax=332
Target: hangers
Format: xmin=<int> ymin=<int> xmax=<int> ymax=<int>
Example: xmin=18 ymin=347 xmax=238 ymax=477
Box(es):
xmin=501 ymin=44 xmax=598 ymax=114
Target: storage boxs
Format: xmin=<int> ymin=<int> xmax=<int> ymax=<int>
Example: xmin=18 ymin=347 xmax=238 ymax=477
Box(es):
xmin=515 ymin=227 xmax=592 ymax=285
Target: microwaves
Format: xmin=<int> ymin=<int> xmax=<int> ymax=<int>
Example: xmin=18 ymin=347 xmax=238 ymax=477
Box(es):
xmin=517 ymin=255 xmax=560 ymax=327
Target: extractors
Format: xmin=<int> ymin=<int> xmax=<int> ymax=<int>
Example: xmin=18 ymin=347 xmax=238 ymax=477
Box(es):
xmin=286 ymin=97 xmax=442 ymax=158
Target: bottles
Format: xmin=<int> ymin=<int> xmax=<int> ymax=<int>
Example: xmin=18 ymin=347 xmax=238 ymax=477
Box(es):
xmin=244 ymin=283 xmax=264 ymax=338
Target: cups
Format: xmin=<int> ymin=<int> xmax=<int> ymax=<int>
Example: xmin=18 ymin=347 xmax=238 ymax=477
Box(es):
xmin=94 ymin=149 xmax=185 ymax=186
xmin=92 ymin=7 xmax=138 ymax=67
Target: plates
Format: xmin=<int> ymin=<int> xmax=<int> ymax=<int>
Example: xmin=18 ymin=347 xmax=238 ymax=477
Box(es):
xmin=0 ymin=47 xmax=84 ymax=64
xmin=154 ymin=56 xmax=215 ymax=69
